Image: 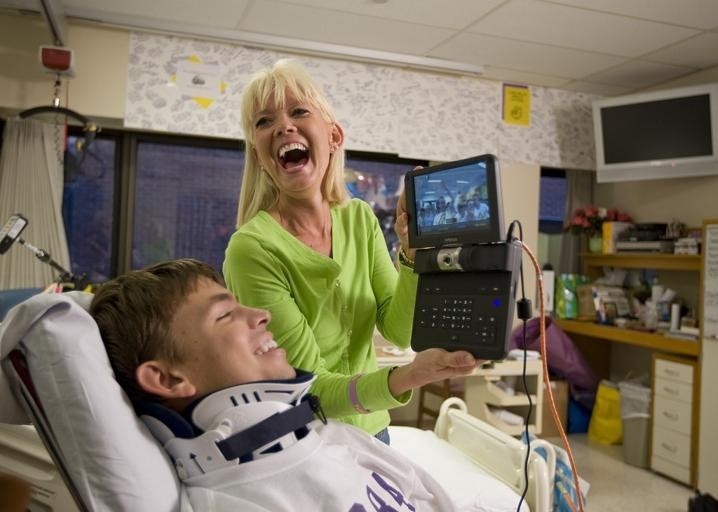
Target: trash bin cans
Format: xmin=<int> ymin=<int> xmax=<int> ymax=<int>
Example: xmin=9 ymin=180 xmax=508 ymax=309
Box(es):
xmin=617 ymin=377 xmax=653 ymax=469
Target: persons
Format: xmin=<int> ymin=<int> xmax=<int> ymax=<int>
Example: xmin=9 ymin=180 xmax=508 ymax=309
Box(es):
xmin=89 ymin=257 xmax=529 ymax=512
xmin=223 ymin=59 xmax=479 ymax=447
xmin=417 ymin=185 xmax=490 ymax=227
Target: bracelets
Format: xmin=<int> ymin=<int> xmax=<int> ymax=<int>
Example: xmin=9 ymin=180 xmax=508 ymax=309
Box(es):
xmin=400 ymin=247 xmax=416 ymax=266
xmin=346 ymin=372 xmax=372 ymax=419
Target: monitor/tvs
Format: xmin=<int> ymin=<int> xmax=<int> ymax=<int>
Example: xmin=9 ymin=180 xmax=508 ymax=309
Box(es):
xmin=591 ymin=83 xmax=718 ymax=185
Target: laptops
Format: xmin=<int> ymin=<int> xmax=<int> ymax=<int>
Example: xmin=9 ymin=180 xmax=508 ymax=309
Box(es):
xmin=403 ymin=153 xmax=523 ymax=359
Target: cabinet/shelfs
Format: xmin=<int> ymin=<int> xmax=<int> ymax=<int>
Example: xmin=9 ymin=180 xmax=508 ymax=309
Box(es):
xmin=692 ymin=220 xmax=718 ymax=503
xmin=417 ymin=349 xmax=542 ymax=440
xmin=545 ymin=216 xmax=698 ymax=492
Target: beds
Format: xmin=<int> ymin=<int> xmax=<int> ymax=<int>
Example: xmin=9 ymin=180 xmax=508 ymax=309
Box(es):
xmin=0 ymin=294 xmax=555 ymax=512
